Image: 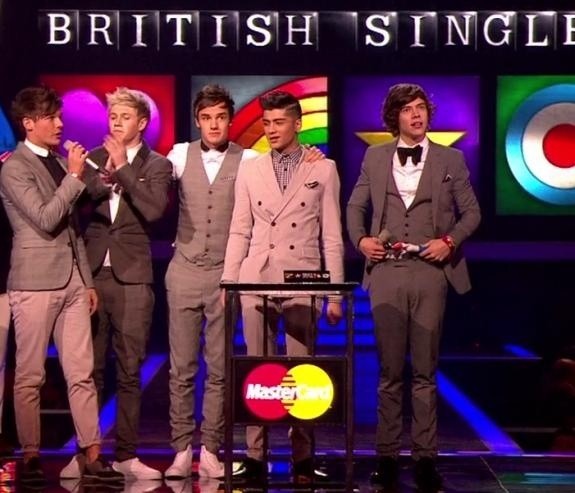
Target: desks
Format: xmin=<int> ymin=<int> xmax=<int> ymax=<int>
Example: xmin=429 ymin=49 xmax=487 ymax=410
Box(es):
xmin=220 ymin=282 xmax=359 ymax=493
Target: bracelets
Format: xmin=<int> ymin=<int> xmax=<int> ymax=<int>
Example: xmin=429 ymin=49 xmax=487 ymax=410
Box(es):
xmin=441 ymin=235 xmax=455 ymax=251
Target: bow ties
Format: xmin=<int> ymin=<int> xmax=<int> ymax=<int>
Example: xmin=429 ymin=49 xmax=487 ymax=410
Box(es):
xmin=395 ymin=145 xmax=423 ymax=167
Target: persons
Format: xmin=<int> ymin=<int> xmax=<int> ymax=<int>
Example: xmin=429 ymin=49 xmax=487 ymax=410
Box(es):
xmin=0 ymin=162 xmax=16 ymax=435
xmin=346 ymin=83 xmax=481 ymax=487
xmin=0 ymin=85 xmax=118 ymax=477
xmin=60 ymin=88 xmax=173 ymax=481
xmin=165 ymin=86 xmax=327 ymax=481
xmin=218 ymin=90 xmax=346 ymax=485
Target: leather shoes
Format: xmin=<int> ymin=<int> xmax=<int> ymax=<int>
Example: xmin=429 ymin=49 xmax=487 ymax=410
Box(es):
xmin=412 ymin=469 xmax=445 ymax=491
xmin=290 ymin=465 xmax=346 ymax=483
xmin=370 ymin=467 xmax=399 ymax=485
xmin=233 ymin=467 xmax=269 ymax=483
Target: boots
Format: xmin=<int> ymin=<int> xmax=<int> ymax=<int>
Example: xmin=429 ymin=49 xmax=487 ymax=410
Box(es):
xmin=199 ymin=445 xmax=225 ymax=478
xmin=165 ymin=444 xmax=193 ymax=479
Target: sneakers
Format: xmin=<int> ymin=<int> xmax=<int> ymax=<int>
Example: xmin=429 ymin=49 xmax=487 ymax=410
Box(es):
xmin=112 ymin=458 xmax=162 ymax=480
xmin=21 ymin=456 xmax=43 ymax=482
xmin=84 ymin=452 xmax=125 ymax=482
xmin=60 ymin=454 xmax=86 ymax=478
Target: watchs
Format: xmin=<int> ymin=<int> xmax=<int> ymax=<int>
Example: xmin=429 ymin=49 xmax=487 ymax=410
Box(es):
xmin=69 ymin=171 xmax=83 ymax=181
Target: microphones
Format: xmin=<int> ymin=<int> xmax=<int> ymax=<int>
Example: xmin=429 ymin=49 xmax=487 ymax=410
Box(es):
xmin=63 ymin=139 xmax=100 ymax=173
xmin=366 ymin=228 xmax=392 ymax=274
